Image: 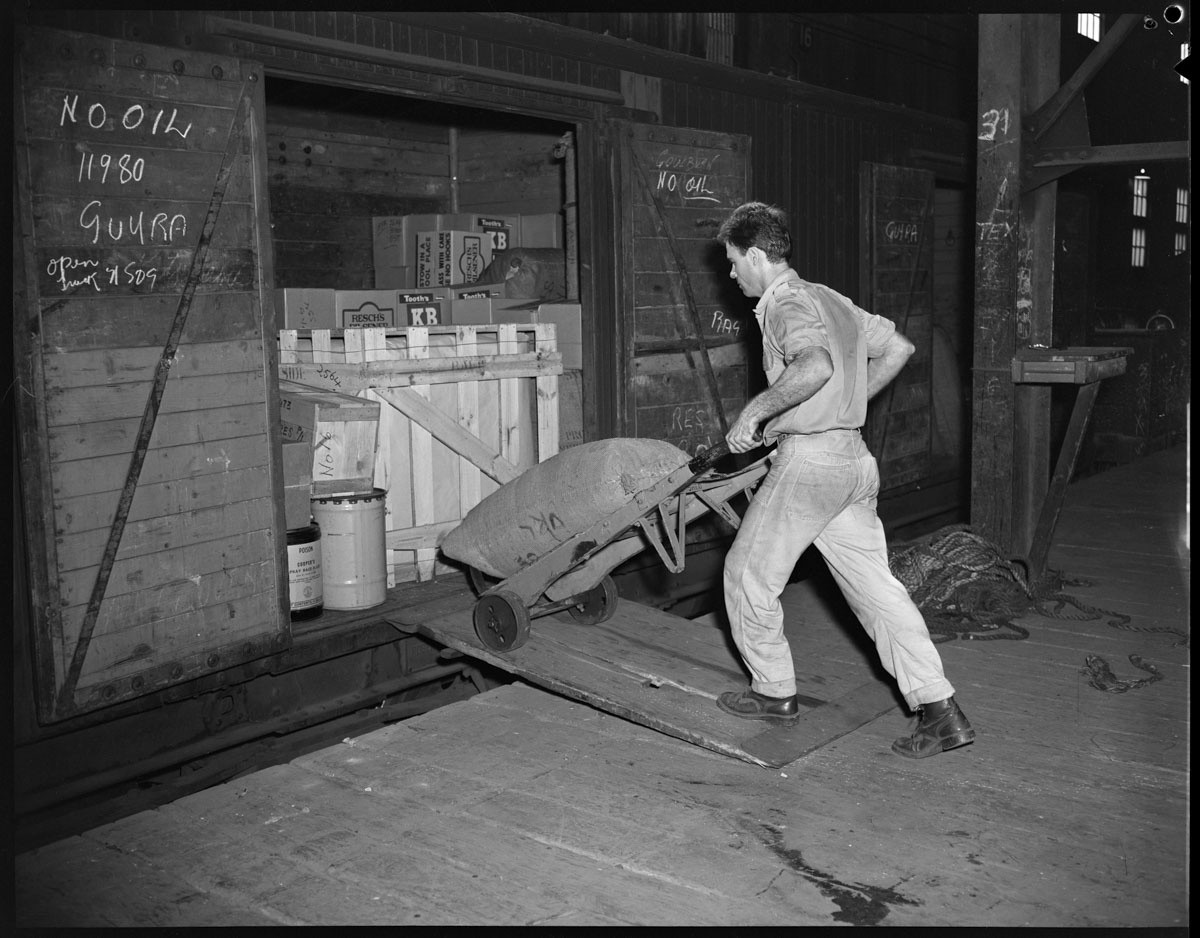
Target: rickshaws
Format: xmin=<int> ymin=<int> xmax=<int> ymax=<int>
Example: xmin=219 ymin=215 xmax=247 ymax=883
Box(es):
xmin=437 ymin=438 xmax=782 ymax=651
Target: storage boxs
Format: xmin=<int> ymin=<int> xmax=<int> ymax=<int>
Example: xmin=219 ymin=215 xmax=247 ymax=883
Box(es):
xmin=276 ymin=322 xmax=563 ymax=588
xmin=275 ymin=212 xmax=582 ymax=371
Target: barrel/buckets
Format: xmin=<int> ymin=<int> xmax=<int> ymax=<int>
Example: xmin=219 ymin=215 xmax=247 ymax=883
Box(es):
xmin=311 ymin=488 xmax=389 ymax=612
xmin=285 ymin=521 xmax=325 ymax=621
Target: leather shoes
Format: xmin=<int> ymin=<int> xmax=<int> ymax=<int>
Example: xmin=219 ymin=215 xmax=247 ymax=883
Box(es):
xmin=892 ymin=695 xmax=976 ymax=760
xmin=715 ymin=688 xmax=800 ymax=729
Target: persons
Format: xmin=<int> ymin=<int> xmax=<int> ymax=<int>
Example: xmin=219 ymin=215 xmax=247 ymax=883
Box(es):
xmin=716 ymin=202 xmax=976 ymax=759
